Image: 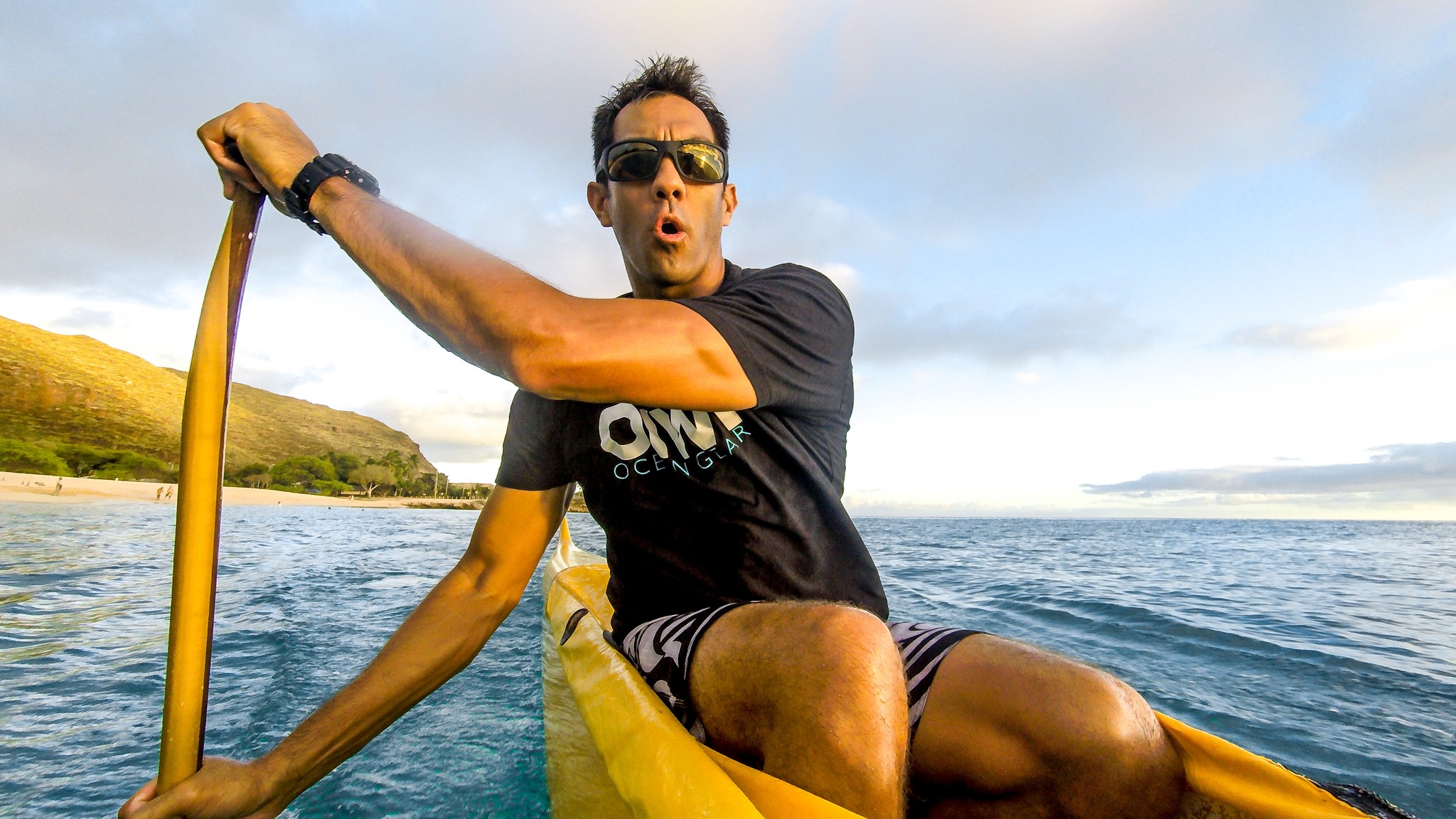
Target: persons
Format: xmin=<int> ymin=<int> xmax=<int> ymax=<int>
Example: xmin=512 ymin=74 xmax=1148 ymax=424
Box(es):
xmin=118 ymin=53 xmax=1183 ymax=819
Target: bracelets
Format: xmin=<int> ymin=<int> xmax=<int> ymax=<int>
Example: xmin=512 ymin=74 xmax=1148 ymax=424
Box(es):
xmin=281 ymin=152 xmax=380 ymax=234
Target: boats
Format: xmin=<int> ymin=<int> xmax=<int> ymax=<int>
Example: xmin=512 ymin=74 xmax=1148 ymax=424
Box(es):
xmin=540 ymin=514 xmax=1387 ymax=819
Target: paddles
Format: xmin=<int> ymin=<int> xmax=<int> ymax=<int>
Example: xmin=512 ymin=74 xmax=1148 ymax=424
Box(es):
xmin=157 ymin=184 xmax=274 ymax=792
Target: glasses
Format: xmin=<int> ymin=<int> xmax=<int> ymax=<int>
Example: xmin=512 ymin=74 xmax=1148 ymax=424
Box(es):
xmin=596 ymin=138 xmax=728 ymax=192
